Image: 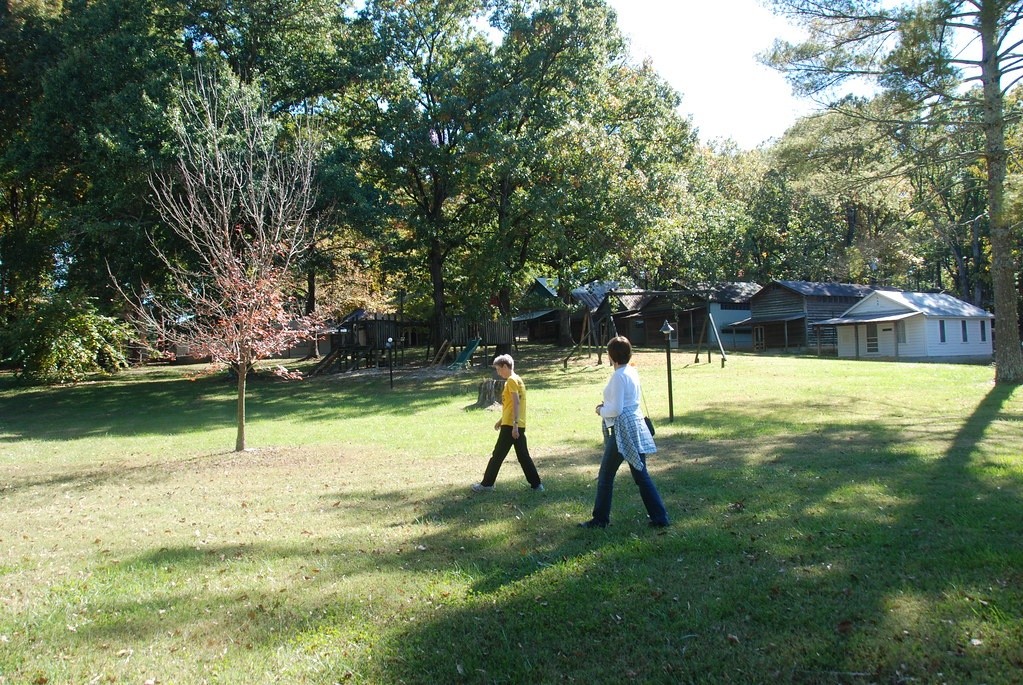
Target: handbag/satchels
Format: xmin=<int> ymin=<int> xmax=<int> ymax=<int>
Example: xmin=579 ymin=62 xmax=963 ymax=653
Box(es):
xmin=644 ymin=416 xmax=655 ymax=436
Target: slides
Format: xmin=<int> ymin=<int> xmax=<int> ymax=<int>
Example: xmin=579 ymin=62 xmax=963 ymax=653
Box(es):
xmin=448 ymin=337 xmax=481 ymax=371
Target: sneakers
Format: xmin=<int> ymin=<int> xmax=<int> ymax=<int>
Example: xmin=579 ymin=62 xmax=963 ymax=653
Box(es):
xmin=471 ymin=484 xmax=493 ymax=494
xmin=527 ymin=484 xmax=545 ymax=494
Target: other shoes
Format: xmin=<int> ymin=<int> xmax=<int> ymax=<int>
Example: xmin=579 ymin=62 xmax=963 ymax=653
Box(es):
xmin=577 ymin=520 xmax=600 ymax=529
xmin=648 ymin=522 xmax=670 ymax=529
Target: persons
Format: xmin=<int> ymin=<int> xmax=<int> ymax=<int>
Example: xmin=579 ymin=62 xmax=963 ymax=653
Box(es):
xmin=577 ymin=335 xmax=671 ymax=529
xmin=472 ymin=354 xmax=545 ymax=494
